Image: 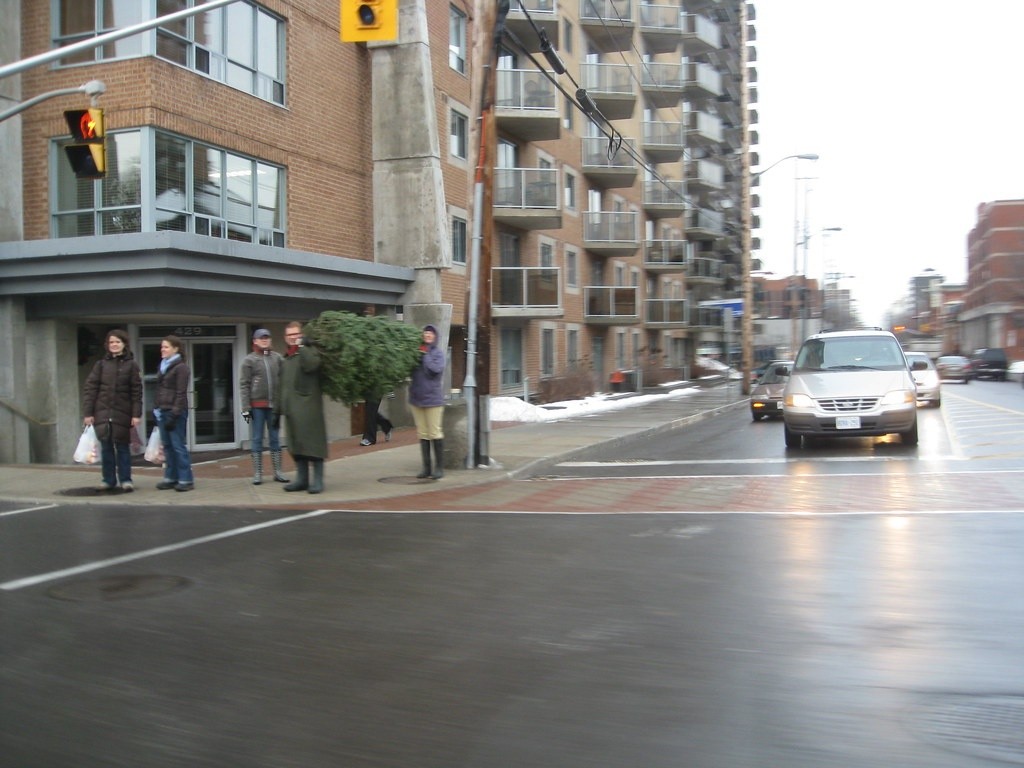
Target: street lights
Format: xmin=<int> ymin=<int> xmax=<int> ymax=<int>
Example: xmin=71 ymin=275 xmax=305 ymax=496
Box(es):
xmin=791 ymin=226 xmax=842 ymax=361
xmin=741 ymin=152 xmax=821 ymax=397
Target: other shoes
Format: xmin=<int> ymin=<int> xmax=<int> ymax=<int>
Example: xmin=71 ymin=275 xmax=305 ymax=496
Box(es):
xmin=97 ymin=481 xmax=112 ymax=490
xmin=156 ymin=481 xmax=178 ymax=489
xmin=120 ymin=481 xmax=134 ymax=492
xmin=175 ymin=482 xmax=194 ymax=491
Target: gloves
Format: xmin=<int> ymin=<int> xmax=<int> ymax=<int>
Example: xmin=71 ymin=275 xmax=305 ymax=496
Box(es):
xmin=242 ymin=411 xmax=254 ymax=423
xmin=270 ymin=414 xmax=279 ymax=430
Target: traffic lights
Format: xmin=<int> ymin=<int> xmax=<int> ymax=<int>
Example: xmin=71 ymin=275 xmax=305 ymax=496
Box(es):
xmin=340 ymin=0 xmax=398 ymax=43
xmin=63 ymin=106 xmax=109 ymax=180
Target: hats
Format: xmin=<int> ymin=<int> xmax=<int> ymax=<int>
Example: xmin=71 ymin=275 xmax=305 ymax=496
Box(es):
xmin=254 ymin=329 xmax=270 ymax=339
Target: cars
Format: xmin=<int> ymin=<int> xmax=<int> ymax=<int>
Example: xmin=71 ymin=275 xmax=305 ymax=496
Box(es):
xmin=902 ymin=351 xmax=942 ymax=409
xmin=934 ymin=355 xmax=978 ymax=385
xmin=749 ymin=359 xmax=795 ymax=422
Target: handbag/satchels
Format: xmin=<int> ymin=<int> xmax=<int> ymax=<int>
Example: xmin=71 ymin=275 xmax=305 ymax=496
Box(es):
xmin=73 ymin=423 xmax=103 ymax=465
xmin=144 ymin=426 xmax=167 ymax=464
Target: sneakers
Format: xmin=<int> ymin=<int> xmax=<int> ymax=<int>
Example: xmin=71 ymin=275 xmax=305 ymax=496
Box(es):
xmin=385 ymin=430 xmax=391 ymax=441
xmin=360 ymin=438 xmax=371 ymax=446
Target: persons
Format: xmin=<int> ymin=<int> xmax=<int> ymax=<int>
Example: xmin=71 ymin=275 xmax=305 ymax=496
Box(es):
xmin=409 ymin=325 xmax=445 ymax=479
xmin=83 ymin=330 xmax=143 ymax=491
xmin=240 ymin=329 xmax=291 ymax=484
xmin=217 ymin=349 xmax=232 ymax=415
xmin=360 ymin=395 xmax=393 ymax=446
xmin=272 ymin=322 xmax=328 ymax=494
xmin=152 ymin=335 xmax=195 ymax=492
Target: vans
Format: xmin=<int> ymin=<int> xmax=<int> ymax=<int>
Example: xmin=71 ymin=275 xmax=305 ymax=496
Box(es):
xmin=774 ymin=324 xmax=929 ymax=450
xmin=970 ymin=347 xmax=1009 ymax=381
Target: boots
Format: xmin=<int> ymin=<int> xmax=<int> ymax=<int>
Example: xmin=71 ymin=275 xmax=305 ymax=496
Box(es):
xmin=270 ymin=451 xmax=290 ymax=482
xmin=284 ymin=460 xmax=309 ymax=491
xmin=308 ymin=461 xmax=323 ymax=493
xmin=418 ymin=439 xmax=431 ymax=479
xmin=431 ymin=439 xmax=443 ymax=479
xmin=251 ymin=453 xmax=262 ymax=485
